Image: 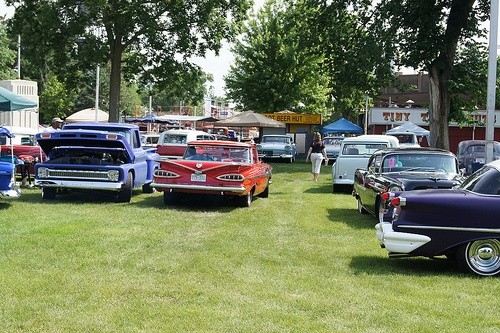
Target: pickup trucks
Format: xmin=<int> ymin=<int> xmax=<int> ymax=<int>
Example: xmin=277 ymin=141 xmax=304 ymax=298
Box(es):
xmin=353 ymin=146 xmax=467 ymax=220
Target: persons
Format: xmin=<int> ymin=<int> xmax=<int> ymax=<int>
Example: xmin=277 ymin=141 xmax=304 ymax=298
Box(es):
xmin=48 ymin=117 xmax=64 ymax=130
xmin=307 ymin=132 xmax=327 ymax=179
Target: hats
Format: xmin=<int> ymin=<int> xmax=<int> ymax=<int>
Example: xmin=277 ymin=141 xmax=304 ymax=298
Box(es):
xmin=52 ymin=117 xmax=64 ymax=123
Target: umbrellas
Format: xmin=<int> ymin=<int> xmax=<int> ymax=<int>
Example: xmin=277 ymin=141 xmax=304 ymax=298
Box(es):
xmin=65 ymin=108 xmax=109 ymax=122
xmin=125 ymin=114 xmax=179 ymax=125
xmin=386 ymin=121 xmax=430 ymax=137
xmin=322 ymin=118 xmax=363 ymax=134
xmin=214 ymin=110 xmax=286 ymax=129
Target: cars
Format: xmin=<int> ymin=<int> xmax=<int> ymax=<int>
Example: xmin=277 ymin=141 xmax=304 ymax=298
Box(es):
xmin=0 ymin=125 xmax=47 ymax=191
xmin=150 ymin=140 xmax=274 ymax=207
xmin=260 ymin=134 xmax=297 ymax=164
xmin=33 ymin=122 xmax=161 ymax=203
xmin=384 ymin=131 xmax=421 ymax=147
xmin=321 ymin=137 xmax=344 ymax=165
xmin=332 ymin=135 xmax=399 ymax=193
xmin=457 ymin=140 xmax=500 ymax=176
xmin=140 ymin=127 xmax=240 ymax=156
xmin=375 ymin=159 xmax=500 ymax=279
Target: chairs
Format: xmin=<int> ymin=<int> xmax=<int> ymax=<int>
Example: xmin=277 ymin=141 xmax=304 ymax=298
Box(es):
xmin=347 ymin=148 xmax=358 ymax=154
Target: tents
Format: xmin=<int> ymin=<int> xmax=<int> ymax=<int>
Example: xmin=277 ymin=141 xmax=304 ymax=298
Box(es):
xmin=0 ymin=85 xmax=44 ymax=197
